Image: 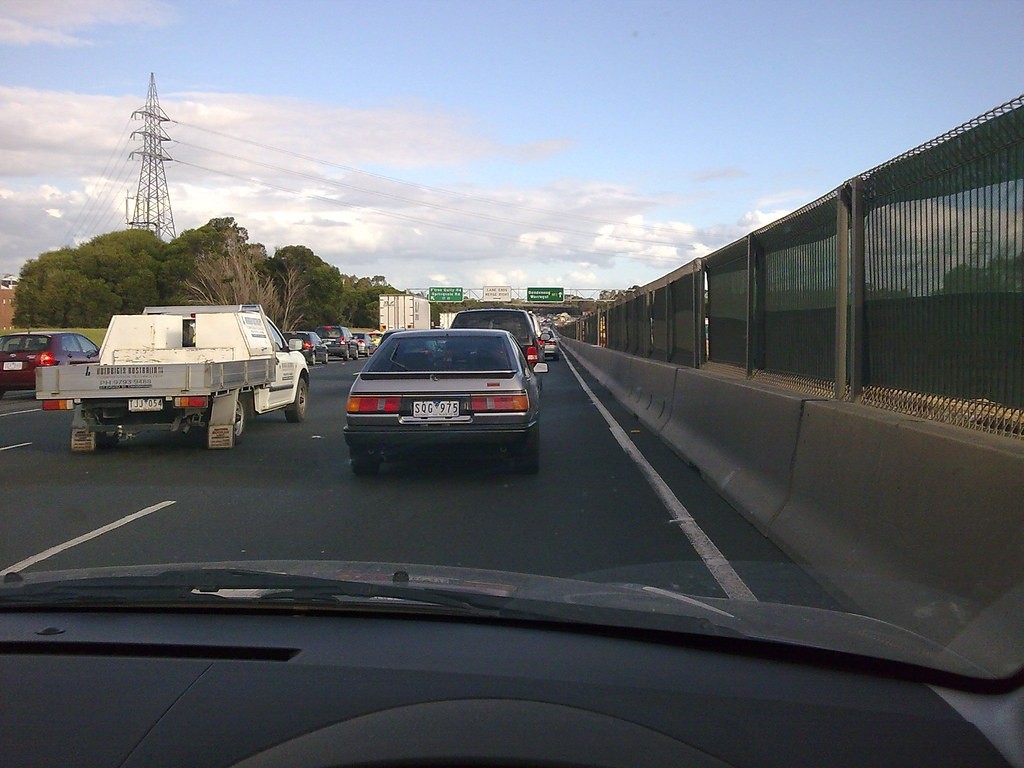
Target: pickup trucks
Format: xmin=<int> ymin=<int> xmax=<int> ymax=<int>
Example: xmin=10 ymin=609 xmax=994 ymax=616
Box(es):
xmin=31 ymin=299 xmax=314 ymax=453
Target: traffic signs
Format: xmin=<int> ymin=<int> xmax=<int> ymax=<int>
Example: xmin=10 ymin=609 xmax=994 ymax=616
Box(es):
xmin=482 ymin=286 xmax=512 ymax=302
xmin=428 ymin=286 xmax=462 ymax=302
xmin=526 ymin=287 xmax=564 ymax=304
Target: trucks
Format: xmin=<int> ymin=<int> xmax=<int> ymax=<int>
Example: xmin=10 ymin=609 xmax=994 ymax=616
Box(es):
xmin=438 ymin=312 xmax=458 ymax=328
xmin=378 ymin=293 xmax=434 ymax=332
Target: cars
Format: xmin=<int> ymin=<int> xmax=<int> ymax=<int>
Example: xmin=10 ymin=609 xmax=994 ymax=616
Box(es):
xmin=0 ymin=330 xmax=102 ymax=400
xmin=342 ymin=327 xmax=549 ymax=477
xmin=366 ymin=329 xmax=384 ymax=347
xmin=527 ymin=311 xmax=571 ymax=361
xmin=281 ymin=331 xmax=329 ymax=365
xmin=352 ymin=331 xmax=372 ymax=357
xmin=3 ymin=561 xmax=1024 ymax=768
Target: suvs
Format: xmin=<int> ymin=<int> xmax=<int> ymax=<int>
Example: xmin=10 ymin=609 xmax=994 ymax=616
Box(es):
xmin=449 ymin=307 xmax=552 ymax=392
xmin=315 ymin=325 xmax=359 ymax=360
